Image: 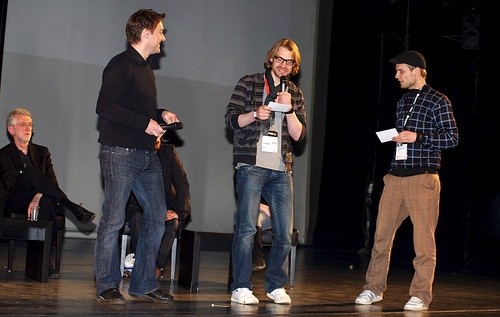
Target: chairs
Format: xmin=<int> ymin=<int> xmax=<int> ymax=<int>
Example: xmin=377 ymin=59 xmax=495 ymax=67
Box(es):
xmin=120 ymin=221 xmax=178 ymax=283
xmin=6 ymin=211 xmax=70 ymax=274
xmin=261 ymin=228 xmax=299 ymax=289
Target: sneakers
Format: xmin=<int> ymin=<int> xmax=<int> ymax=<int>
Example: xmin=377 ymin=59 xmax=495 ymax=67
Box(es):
xmin=266 ymin=287 xmax=291 ymax=304
xmin=403 ymin=296 xmax=428 ymax=311
xmin=355 ymin=290 xmax=383 ymax=305
xmin=230 ymin=287 xmax=259 ymax=305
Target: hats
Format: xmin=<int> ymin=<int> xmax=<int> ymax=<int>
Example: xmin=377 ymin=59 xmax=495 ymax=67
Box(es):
xmin=388 ymin=51 xmax=426 ymax=69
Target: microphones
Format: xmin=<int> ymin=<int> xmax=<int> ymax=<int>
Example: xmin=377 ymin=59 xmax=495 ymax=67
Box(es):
xmin=161 ymin=121 xmax=183 ymax=130
xmin=280 ymin=75 xmax=287 ymax=92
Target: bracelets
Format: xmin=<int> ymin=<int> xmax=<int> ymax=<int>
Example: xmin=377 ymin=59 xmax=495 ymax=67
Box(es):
xmin=254 ymin=110 xmax=261 ymax=120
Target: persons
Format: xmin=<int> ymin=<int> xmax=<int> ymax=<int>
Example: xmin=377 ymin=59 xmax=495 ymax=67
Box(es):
xmin=91 ymin=9 xmax=179 ymax=303
xmin=126 ymin=146 xmax=191 ymax=279
xmin=0 ymin=107 xmax=97 ymax=279
xmin=354 ymin=51 xmax=459 ymax=311
xmin=225 ymin=38 xmax=307 ymax=304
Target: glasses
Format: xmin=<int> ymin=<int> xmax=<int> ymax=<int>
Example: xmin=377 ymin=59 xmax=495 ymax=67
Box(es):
xmin=9 ymin=122 xmax=34 ymax=127
xmin=273 ymin=56 xmax=296 ymax=66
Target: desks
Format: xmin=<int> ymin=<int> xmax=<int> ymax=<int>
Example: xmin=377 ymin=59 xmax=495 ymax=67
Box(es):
xmin=177 ymin=228 xmax=234 ymax=294
xmin=0 ymin=217 xmax=54 ymax=284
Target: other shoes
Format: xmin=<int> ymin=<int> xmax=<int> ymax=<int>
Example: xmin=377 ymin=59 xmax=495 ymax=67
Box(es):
xmin=48 ymin=264 xmax=59 ymax=279
xmin=74 ymin=206 xmax=96 ymax=224
xmin=154 ymin=268 xmax=162 ymax=280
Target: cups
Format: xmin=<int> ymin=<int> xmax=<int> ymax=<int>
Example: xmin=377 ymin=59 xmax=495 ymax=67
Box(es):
xmin=30 ymin=206 xmax=40 ymax=221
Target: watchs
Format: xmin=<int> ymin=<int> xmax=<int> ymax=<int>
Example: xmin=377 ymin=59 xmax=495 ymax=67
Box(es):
xmin=284 ymin=108 xmax=294 ymax=114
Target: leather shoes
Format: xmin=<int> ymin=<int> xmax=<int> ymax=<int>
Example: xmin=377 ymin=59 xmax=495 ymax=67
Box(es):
xmin=99 ymin=288 xmax=126 ymax=305
xmin=128 ymin=289 xmax=174 ymax=303
